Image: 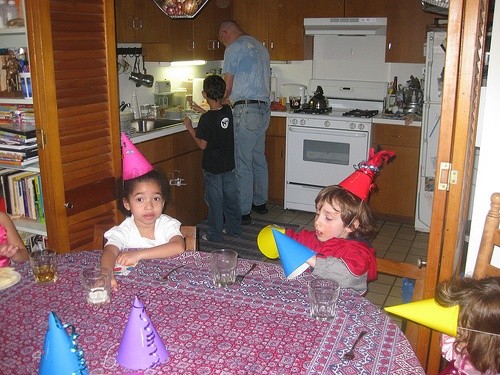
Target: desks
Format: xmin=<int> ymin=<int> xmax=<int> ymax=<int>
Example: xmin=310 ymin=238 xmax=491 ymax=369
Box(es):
xmin=0 ymin=248 xmax=426 ymax=375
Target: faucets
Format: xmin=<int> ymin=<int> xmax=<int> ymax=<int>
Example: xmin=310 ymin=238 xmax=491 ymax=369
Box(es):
xmin=119 ymin=102 xmax=131 ymax=111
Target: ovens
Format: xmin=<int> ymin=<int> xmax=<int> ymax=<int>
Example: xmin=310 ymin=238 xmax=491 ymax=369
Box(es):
xmin=287 ymin=127 xmax=371 ymax=215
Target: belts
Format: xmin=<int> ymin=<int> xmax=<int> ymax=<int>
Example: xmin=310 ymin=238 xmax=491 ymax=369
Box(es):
xmin=232 ymin=100 xmax=266 ymax=109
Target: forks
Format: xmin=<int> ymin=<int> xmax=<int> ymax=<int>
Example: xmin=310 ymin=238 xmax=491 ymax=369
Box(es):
xmin=235 ymin=263 xmax=257 ymax=285
xmin=342 ymin=331 xmax=367 ymax=360
xmin=160 ymin=267 xmax=182 ymax=282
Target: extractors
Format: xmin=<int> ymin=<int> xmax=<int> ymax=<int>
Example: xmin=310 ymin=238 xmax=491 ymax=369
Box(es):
xmin=304 ymin=17 xmax=387 ymax=35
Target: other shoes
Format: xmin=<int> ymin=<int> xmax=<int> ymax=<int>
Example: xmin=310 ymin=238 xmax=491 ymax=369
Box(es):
xmin=223 ymin=228 xmax=227 ymax=234
xmin=252 ymin=203 xmax=268 ymax=214
xmin=202 ymin=233 xmax=208 ymax=241
xmin=241 ymin=214 xmax=251 ymax=225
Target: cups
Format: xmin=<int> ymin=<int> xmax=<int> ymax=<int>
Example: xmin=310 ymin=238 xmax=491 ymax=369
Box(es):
xmin=19 ymin=73 xmax=32 ymax=99
xmin=307 ymin=279 xmax=341 ymax=321
xmin=83 ymin=269 xmax=112 ymax=306
xmin=30 ymin=249 xmax=57 ymax=284
xmin=289 ymin=96 xmax=301 ymax=109
xmin=210 ymin=248 xmax=238 ymax=287
xmin=118 ymin=58 xmax=132 ymax=73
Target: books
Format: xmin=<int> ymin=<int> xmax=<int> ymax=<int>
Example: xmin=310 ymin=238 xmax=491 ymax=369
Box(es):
xmin=0 ymin=103 xmax=48 ymax=254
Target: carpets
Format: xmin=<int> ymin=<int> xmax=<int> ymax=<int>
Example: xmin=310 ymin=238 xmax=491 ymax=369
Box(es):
xmin=196 ymin=219 xmax=299 ymax=262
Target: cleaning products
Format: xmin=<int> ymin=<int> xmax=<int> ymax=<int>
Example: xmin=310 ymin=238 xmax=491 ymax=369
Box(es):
xmin=151 ymin=105 xmax=160 ymax=118
xmin=131 ymin=89 xmax=140 ymax=120
xmin=140 ymin=103 xmax=151 ymax=120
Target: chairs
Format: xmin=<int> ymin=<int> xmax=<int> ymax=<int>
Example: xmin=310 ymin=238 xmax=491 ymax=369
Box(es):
xmin=92 ymin=224 xmax=198 ymax=252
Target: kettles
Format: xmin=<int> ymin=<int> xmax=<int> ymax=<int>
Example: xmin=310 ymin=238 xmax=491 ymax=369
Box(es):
xmin=308 ymin=86 xmax=329 ymax=109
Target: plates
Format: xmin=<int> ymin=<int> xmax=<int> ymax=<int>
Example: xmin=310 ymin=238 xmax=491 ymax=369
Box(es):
xmin=0 ymin=269 xmax=21 ymax=290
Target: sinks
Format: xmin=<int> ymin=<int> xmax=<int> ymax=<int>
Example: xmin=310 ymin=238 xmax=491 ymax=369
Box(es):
xmin=127 ymin=119 xmax=184 ymax=132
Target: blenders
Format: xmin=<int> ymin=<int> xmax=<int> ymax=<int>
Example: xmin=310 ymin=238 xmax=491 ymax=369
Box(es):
xmin=403 ymin=88 xmax=423 ymax=115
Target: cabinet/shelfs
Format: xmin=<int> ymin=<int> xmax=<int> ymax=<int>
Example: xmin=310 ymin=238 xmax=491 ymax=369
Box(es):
xmin=384 ymin=0 xmax=438 ymax=64
xmin=0 ymin=0 xmax=47 ymax=237
xmin=141 ymin=20 xmax=193 ymax=63
xmin=231 ymin=0 xmax=313 ymax=61
xmin=193 ymin=0 xmax=232 ymax=61
xmin=134 ymin=128 xmax=209 ymax=226
xmin=265 ymin=117 xmax=286 ymax=206
xmin=368 ymin=123 xmax=422 ymax=225
xmin=113 ymin=0 xmax=171 ymax=44
xmin=303 ymin=0 xmax=388 ymax=18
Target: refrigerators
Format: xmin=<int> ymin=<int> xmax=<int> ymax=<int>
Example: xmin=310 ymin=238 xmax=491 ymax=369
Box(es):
xmin=415 ymin=31 xmax=447 ymax=233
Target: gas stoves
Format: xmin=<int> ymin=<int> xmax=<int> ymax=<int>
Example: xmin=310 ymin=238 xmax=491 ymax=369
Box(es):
xmin=289 ymin=107 xmax=379 ymax=132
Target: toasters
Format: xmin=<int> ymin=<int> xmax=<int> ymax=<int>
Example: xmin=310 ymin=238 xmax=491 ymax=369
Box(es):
xmin=386 ymin=92 xmax=403 ymax=114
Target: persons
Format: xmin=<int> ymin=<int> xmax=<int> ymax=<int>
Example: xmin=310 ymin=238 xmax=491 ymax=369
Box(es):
xmin=94 ymin=132 xmax=185 ymax=291
xmin=384 ymin=275 xmax=500 ymax=375
xmin=0 ymin=211 xmax=27 ymax=269
xmin=218 ymin=20 xmax=271 ymax=224
xmin=183 ymin=74 xmax=242 ymax=241
xmin=258 ymin=148 xmax=397 ymax=296
